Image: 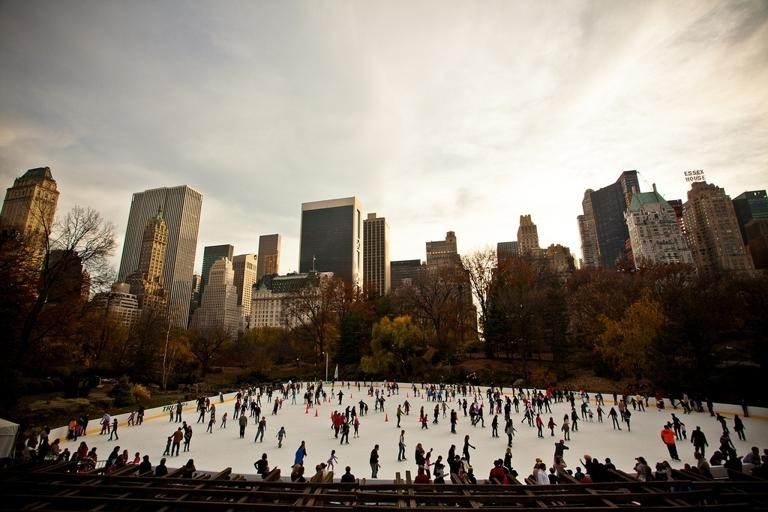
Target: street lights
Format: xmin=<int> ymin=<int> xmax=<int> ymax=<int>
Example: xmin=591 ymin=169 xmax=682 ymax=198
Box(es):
xmin=163 ymin=319 xmax=174 ymax=391
xmin=321 ymin=351 xmax=328 ymax=383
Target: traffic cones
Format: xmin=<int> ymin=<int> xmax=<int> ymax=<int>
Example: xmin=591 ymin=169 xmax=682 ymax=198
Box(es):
xmin=309 ymin=402 xmax=311 ymax=406
xmin=327 ymin=396 xmax=331 ymax=403
xmin=313 ymin=408 xmax=319 ymax=417
xmin=306 ymin=403 xmax=309 ymax=410
xmin=350 ymin=393 xmax=353 ymax=398
xmin=421 ymin=394 xmax=424 ymax=399
xmin=305 ymin=407 xmax=309 ymax=415
xmin=481 ymin=401 xmax=484 ymax=406
xmin=329 ymin=411 xmax=333 ymax=419
xmin=406 ymin=392 xmax=408 ymax=397
xmin=449 ymin=396 xmax=452 ymax=402
xmin=317 ymin=399 xmax=321 ymax=405
xmin=385 ymin=413 xmax=388 ymax=421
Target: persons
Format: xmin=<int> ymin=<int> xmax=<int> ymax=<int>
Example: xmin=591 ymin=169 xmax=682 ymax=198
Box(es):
xmin=14 ymin=376 xmax=768 ymax=488
